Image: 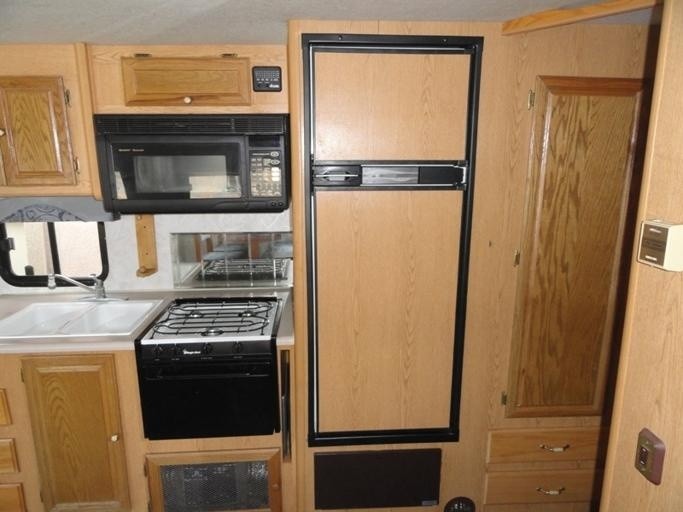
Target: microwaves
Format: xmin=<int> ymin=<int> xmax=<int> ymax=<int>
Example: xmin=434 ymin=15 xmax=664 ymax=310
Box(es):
xmin=90 ymin=112 xmax=289 ymax=214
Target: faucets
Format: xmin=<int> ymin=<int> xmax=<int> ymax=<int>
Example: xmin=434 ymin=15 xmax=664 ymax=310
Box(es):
xmin=54 ymin=274 xmax=106 ymax=299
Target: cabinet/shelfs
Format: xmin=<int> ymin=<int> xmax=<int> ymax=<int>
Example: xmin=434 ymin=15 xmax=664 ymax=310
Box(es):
xmin=500 ymin=75 xmax=643 ymax=419
xmin=0 ymin=43 xmax=100 ymax=201
xmin=480 ymin=422 xmax=601 ymax=510
xmin=84 ymin=45 xmax=287 ymax=118
xmin=301 ymin=32 xmax=482 ymax=448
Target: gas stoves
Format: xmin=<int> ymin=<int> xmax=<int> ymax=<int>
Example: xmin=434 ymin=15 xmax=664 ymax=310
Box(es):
xmin=135 ymin=299 xmax=281 ymax=440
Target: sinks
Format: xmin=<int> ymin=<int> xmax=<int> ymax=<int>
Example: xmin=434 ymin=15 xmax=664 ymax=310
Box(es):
xmin=1 ymin=301 xmax=92 ymax=336
xmin=62 ymin=299 xmax=157 ymax=337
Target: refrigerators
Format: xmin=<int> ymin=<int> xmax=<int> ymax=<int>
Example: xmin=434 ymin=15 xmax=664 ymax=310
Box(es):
xmin=310 ymin=41 xmax=471 ymax=434
